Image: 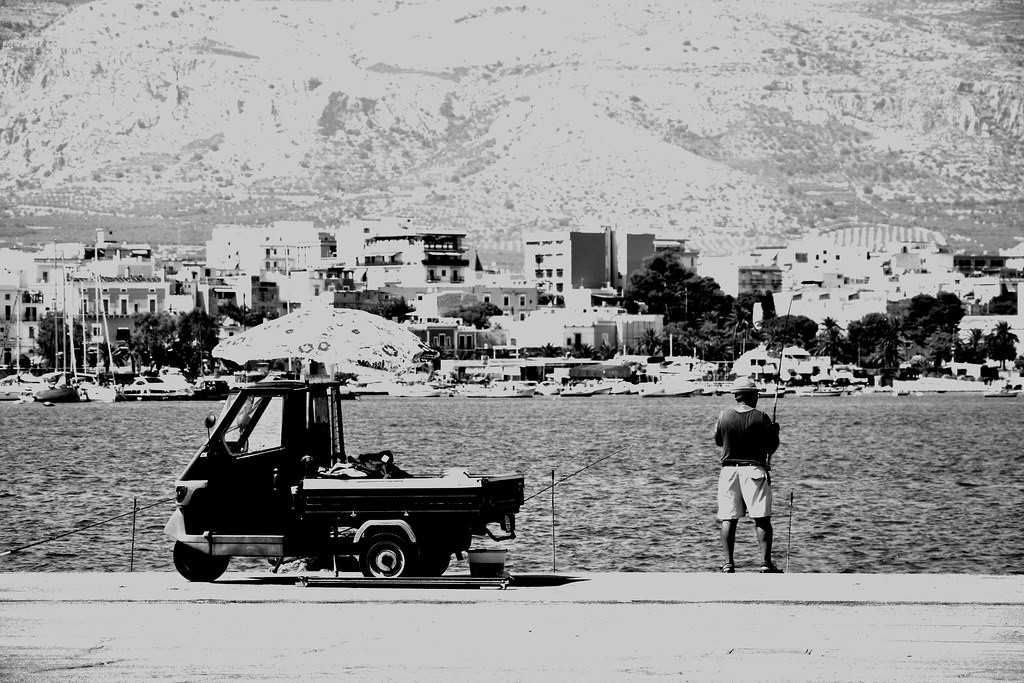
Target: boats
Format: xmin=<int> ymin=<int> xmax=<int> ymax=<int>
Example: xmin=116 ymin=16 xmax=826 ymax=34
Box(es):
xmin=32 ymin=242 xmax=78 ymax=402
xmin=388 ymin=379 xmax=442 ymax=398
xmin=537 ymin=376 xmax=639 ymax=396
xmin=705 ymin=378 xmax=786 ymax=397
xmin=984 ymin=378 xmax=1018 ymax=398
xmin=800 ymin=387 xmax=842 ymax=397
xmin=0 ymin=290 xmax=41 ymax=399
xmin=116 ymin=375 xmax=229 ymax=402
xmin=79 ymin=243 xmax=117 ymax=403
xmin=638 ymin=379 xmax=704 ymax=398
xmin=454 ymin=376 xmax=535 ymax=396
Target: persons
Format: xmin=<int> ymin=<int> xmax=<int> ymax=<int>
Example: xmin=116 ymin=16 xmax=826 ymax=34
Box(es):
xmin=714 ymin=377 xmax=784 ymax=573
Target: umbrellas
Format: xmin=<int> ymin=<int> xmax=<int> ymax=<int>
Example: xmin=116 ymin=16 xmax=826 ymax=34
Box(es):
xmin=212 ymin=303 xmax=436 ymax=578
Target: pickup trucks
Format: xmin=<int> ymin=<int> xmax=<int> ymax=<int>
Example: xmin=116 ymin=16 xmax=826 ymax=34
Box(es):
xmin=160 ymin=385 xmax=530 ymax=587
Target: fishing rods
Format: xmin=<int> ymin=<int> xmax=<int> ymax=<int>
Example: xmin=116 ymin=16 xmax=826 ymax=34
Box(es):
xmin=764 ymin=288 xmax=796 ymax=487
xmin=524 ymin=440 xmax=635 ymax=504
xmin=0 ymin=496 xmax=177 ymax=558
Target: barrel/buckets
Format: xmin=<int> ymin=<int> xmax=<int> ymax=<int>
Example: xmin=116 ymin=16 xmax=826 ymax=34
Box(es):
xmin=465 ymin=549 xmax=508 ymax=581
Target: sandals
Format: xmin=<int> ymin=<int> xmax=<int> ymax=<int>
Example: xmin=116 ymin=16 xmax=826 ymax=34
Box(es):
xmin=721 ymin=564 xmax=734 ymax=573
xmin=760 ymin=561 xmax=783 ymax=573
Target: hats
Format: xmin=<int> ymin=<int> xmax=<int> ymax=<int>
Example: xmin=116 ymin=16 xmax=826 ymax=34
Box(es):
xmin=730 ymin=378 xmax=763 ymax=393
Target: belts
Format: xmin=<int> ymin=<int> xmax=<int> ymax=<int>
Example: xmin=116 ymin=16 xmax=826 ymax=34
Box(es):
xmin=723 ymin=461 xmax=756 ymax=467
